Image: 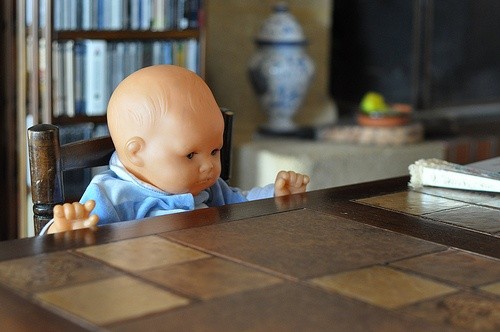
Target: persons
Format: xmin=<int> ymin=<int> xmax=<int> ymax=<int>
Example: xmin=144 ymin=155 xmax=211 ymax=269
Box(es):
xmin=38 ymin=64 xmax=309 ymax=237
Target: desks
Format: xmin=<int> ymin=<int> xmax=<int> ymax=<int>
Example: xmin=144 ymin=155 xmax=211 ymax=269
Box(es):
xmin=0 ymin=171 xmax=500 ymax=332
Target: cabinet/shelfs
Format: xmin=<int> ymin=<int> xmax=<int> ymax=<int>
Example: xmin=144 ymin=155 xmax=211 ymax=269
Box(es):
xmin=0 ymin=0 xmax=209 ymax=242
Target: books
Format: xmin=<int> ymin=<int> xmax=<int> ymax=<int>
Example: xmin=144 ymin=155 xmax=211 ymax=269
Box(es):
xmin=25 ymin=116 xmax=112 ymax=201
xmin=25 ymin=39 xmax=203 ymax=116
xmin=21 ymin=0 xmax=201 ymax=32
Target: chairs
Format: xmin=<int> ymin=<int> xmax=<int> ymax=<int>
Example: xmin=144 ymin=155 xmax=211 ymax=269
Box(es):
xmin=27 ymin=107 xmax=234 ymax=238
